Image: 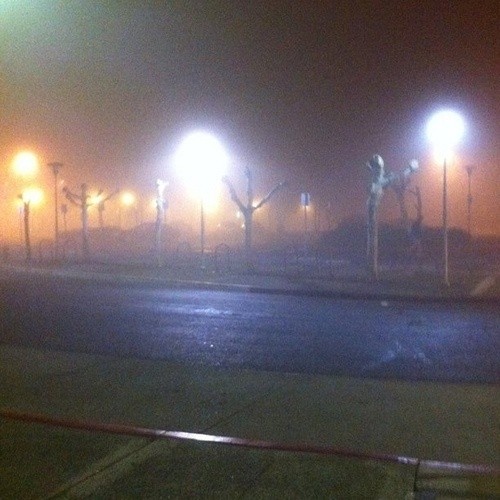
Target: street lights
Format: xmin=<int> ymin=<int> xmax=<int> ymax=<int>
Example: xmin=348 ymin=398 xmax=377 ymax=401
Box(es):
xmin=463 ymin=163 xmax=478 ymax=234
xmin=47 ymin=161 xmax=66 ymax=268
